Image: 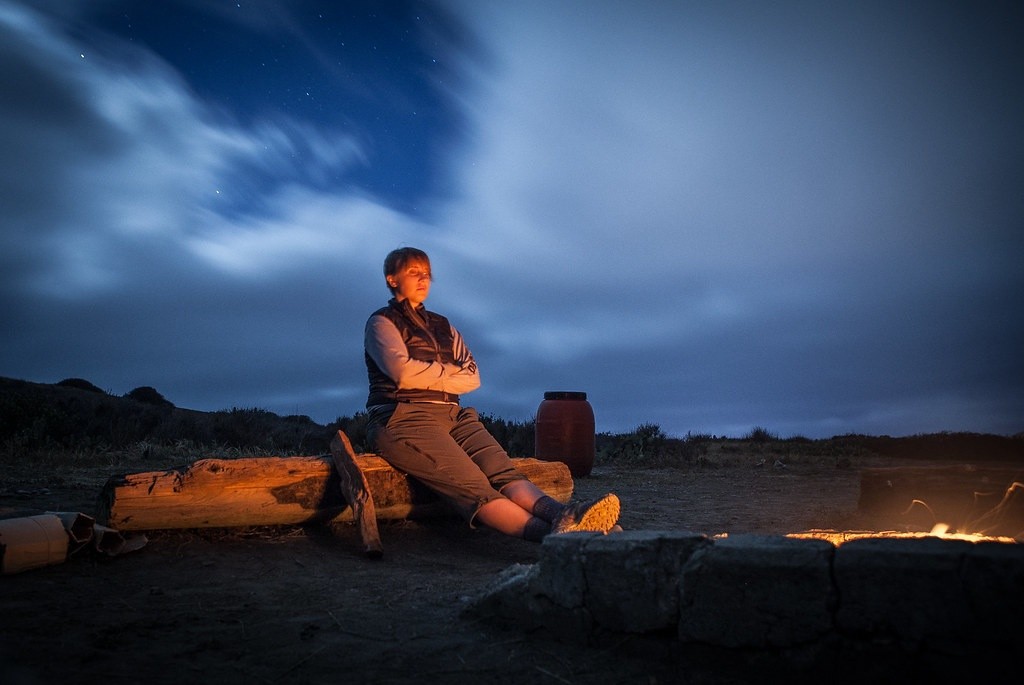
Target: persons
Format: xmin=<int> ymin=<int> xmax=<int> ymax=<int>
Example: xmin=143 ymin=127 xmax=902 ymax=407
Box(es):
xmin=363 ymin=247 xmax=622 ymax=544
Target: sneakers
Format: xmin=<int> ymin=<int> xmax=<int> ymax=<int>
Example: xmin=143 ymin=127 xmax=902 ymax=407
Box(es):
xmin=552 ymin=494 xmax=623 ymax=532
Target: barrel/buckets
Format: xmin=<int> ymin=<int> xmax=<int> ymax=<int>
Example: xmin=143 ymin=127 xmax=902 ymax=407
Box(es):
xmin=535 ymin=392 xmax=595 ymax=477
